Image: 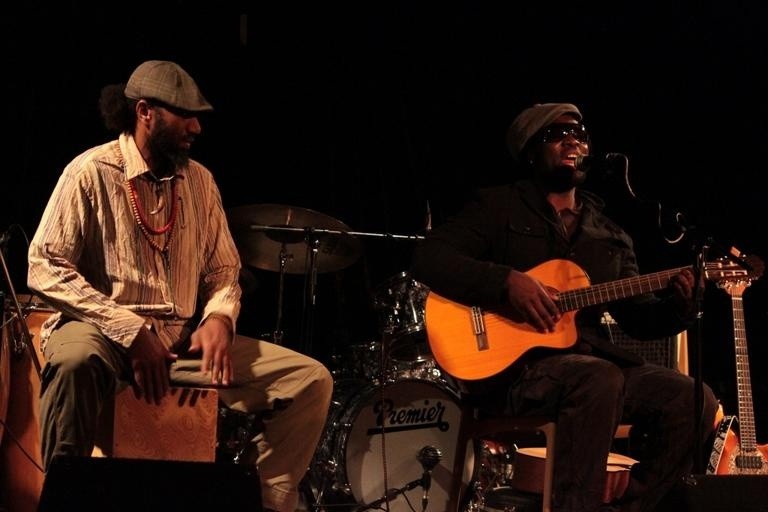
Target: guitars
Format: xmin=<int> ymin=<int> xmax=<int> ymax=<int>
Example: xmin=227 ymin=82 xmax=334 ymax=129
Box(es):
xmin=706 ymin=247 xmax=768 ymax=476
xmin=426 ymin=248 xmax=747 ymax=382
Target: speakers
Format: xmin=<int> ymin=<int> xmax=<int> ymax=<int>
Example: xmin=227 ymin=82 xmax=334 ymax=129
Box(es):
xmin=37 ymin=454 xmax=263 ymax=512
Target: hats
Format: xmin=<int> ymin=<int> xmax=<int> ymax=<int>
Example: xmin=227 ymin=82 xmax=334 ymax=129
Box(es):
xmin=510 ymin=102 xmax=582 ymax=154
xmin=124 ymin=59 xmax=213 ymax=112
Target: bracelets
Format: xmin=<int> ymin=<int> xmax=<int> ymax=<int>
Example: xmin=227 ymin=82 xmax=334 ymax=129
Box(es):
xmin=206 ymin=313 xmax=232 ymax=335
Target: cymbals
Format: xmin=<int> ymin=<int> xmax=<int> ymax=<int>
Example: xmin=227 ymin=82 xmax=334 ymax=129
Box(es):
xmin=227 ymin=204 xmax=356 ymax=273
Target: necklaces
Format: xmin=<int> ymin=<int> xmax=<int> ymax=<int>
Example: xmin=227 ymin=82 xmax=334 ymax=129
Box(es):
xmin=110 ymin=142 xmax=181 ymax=252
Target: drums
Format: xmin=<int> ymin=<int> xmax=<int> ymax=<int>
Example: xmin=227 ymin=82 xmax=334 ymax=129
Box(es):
xmin=324 ymin=361 xmax=480 ymax=512
xmin=10 ymin=291 xmax=62 ymax=510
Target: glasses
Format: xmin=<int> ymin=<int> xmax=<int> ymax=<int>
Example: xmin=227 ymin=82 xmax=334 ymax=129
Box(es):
xmin=532 ymin=123 xmax=588 ymax=144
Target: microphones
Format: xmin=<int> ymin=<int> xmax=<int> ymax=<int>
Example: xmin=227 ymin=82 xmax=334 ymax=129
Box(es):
xmin=417 ymin=445 xmax=442 ymax=512
xmin=574 ymin=153 xmax=621 ymax=171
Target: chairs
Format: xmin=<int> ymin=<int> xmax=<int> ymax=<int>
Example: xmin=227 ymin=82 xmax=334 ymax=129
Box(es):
xmin=445 ymin=325 xmax=691 ymax=511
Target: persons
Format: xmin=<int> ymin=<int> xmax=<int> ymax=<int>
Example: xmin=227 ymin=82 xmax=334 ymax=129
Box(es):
xmin=398 ymin=100 xmax=720 ymax=511
xmin=25 ymin=57 xmax=337 ymax=511
